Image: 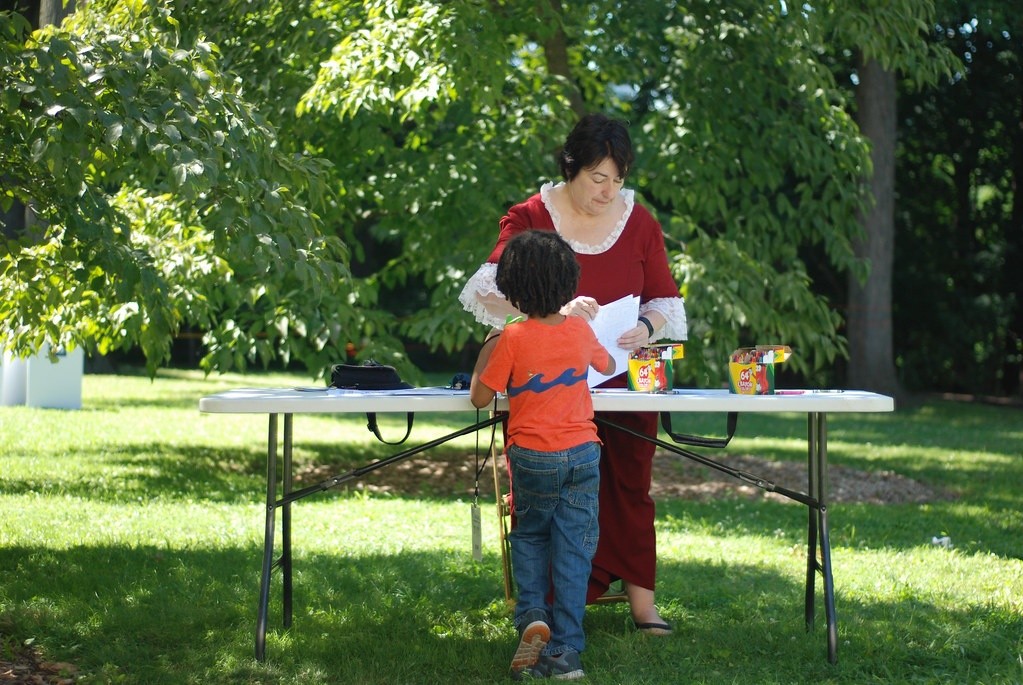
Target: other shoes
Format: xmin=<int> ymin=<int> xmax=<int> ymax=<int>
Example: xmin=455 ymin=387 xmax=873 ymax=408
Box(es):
xmin=635 ymin=620 xmax=672 ymax=635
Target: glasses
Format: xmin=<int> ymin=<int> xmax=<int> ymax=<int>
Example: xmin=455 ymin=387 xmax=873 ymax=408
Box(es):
xmin=361 ymin=357 xmax=382 ymax=366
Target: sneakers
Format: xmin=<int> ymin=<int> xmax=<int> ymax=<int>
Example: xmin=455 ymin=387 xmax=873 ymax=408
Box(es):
xmin=507 ymin=607 xmax=552 ymax=680
xmin=533 ymin=650 xmax=585 ymax=680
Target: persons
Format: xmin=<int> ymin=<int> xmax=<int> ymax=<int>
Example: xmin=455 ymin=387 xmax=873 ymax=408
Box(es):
xmin=469 ymin=230 xmax=617 ymax=683
xmin=483 ymin=114 xmax=688 ymax=636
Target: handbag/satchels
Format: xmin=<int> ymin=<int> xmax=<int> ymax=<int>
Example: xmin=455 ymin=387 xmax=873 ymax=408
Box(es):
xmin=330 ymin=363 xmax=401 ymax=390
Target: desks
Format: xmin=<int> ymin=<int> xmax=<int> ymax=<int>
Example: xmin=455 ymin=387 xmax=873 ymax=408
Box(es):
xmin=199 ymin=388 xmax=895 ymax=665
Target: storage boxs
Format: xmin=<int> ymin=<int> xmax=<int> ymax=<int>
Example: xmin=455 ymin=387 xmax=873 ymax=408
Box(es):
xmin=729 ymin=344 xmax=791 ymax=395
xmin=627 ymin=343 xmax=685 ymax=393
